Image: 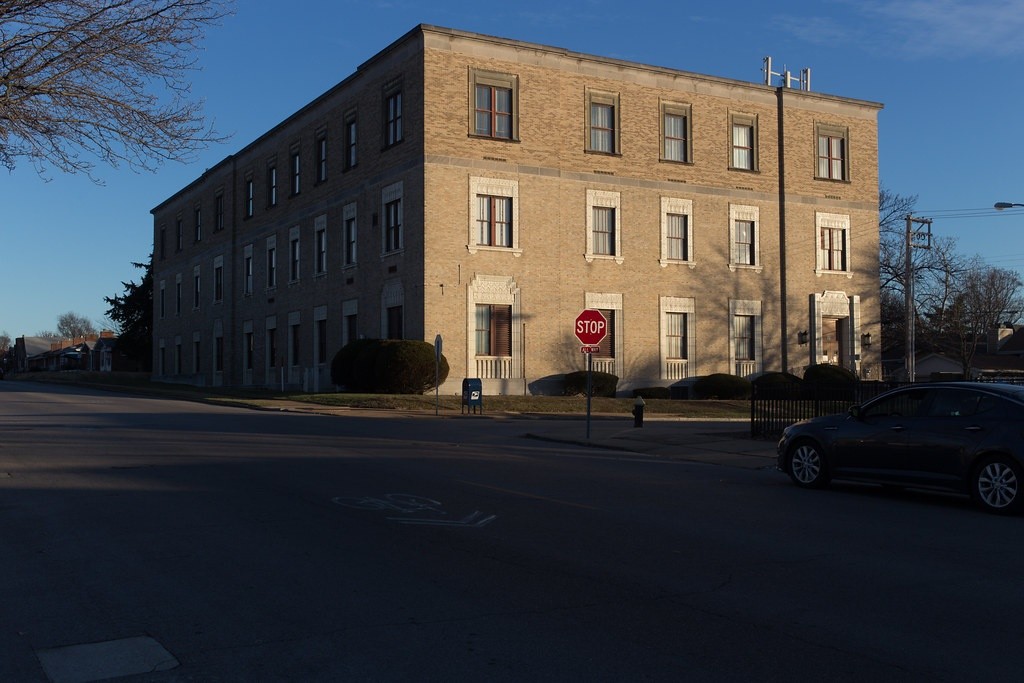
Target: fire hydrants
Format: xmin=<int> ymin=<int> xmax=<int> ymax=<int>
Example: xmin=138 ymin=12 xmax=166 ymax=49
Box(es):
xmin=632 ymin=396 xmax=646 ymax=427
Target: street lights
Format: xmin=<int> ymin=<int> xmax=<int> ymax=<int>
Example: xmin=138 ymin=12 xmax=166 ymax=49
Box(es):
xmin=994 ymin=202 xmax=1024 ymax=210
xmin=761 ymin=55 xmax=811 ymax=379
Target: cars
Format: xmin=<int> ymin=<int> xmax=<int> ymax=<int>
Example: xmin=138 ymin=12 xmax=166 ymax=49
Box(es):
xmin=776 ymin=382 xmax=1024 ymax=515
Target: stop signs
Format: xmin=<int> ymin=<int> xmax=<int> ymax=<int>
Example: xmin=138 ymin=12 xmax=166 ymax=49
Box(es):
xmin=574 ymin=309 xmax=607 ymax=345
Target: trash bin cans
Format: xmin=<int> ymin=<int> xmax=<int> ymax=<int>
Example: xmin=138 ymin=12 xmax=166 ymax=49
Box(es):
xmin=461 ymin=378 xmax=482 ymax=415
